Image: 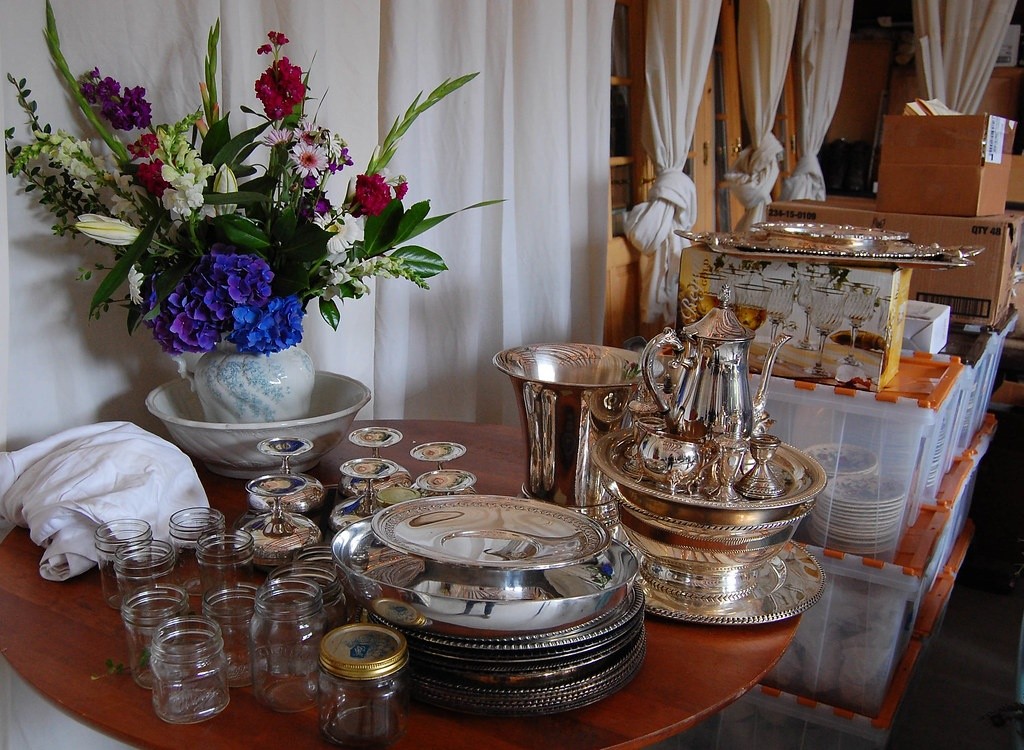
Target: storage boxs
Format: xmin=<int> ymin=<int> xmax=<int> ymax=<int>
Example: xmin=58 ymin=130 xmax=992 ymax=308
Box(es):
xmin=627 ymin=38 xmax=1023 ymax=750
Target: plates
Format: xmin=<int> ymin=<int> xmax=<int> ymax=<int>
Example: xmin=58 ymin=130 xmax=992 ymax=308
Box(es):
xmin=633 ymin=541 xmax=827 ymax=626
xmin=356 ymin=581 xmax=648 ymax=719
xmin=802 ymin=380 xmax=978 ymax=555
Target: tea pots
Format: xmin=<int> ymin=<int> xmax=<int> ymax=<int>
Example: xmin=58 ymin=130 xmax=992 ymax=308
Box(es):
xmin=640 ymin=286 xmax=793 ymax=446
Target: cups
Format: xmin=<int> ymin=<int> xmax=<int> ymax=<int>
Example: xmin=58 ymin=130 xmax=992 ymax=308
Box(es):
xmin=625 ymin=400 xmax=790 ymax=501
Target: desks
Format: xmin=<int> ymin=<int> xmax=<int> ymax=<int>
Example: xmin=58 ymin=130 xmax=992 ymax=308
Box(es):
xmin=0 ymin=423 xmax=801 ymax=750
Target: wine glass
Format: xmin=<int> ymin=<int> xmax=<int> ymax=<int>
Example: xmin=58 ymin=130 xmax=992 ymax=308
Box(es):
xmin=237 ymin=426 xmax=477 ymax=565
xmin=693 ymin=268 xmax=881 ymax=380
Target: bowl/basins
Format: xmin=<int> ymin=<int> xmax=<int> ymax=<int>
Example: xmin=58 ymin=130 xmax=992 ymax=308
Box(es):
xmin=331 ymin=494 xmax=639 ymax=631
xmin=591 ymin=425 xmax=829 ymax=604
xmin=145 ymin=370 xmax=372 ymax=482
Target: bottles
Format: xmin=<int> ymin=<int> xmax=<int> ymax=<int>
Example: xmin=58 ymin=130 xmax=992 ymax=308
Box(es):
xmin=493 ymin=341 xmax=666 ymax=536
xmin=93 ymin=507 xmax=409 ymax=750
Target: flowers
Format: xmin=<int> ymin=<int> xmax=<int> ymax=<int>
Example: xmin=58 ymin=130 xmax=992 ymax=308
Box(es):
xmin=0 ymin=0 xmax=509 ymax=356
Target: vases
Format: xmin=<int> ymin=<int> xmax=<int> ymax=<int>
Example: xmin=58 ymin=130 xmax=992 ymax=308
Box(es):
xmin=193 ymin=344 xmax=315 ymax=424
